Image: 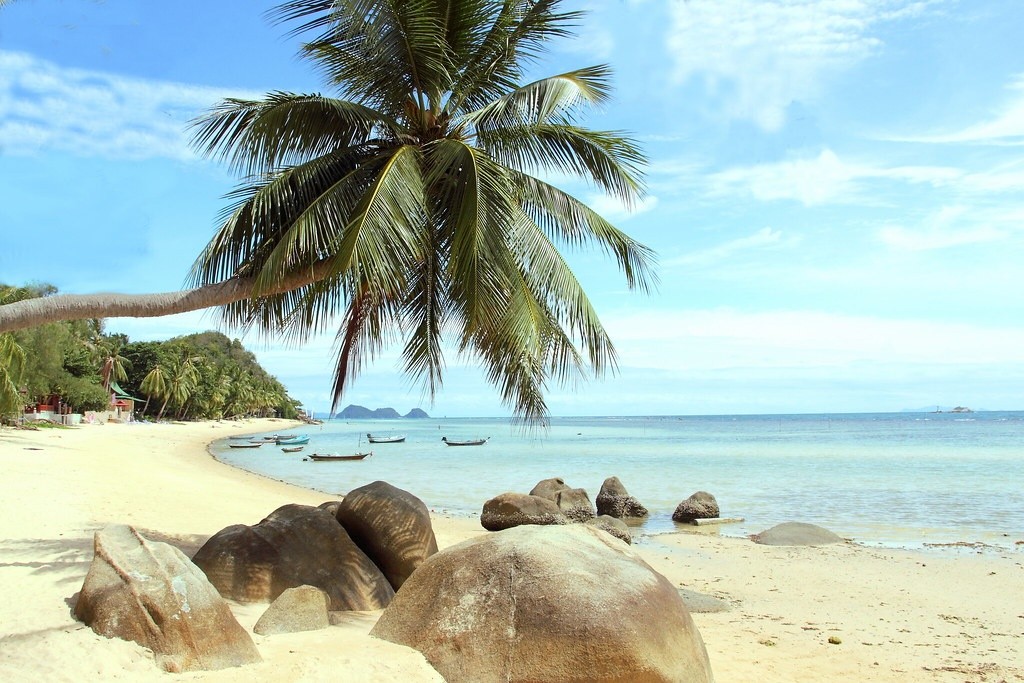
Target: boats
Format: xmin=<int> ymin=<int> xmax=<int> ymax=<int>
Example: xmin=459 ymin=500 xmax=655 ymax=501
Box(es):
xmin=367 ymin=433 xmax=406 ymax=443
xmin=281 ymin=447 xmax=303 ymax=452
xmin=230 ymin=444 xmax=261 ymax=448
xmin=229 ymin=436 xmax=253 ymax=439
xmin=275 ymin=436 xmax=309 ymax=444
xmin=442 ymin=437 xmax=489 ymax=446
xmin=264 ymin=436 xmax=295 ymax=439
xmin=249 ymin=439 xmax=275 ymax=443
xmin=307 ymin=453 xmax=368 ymax=460
